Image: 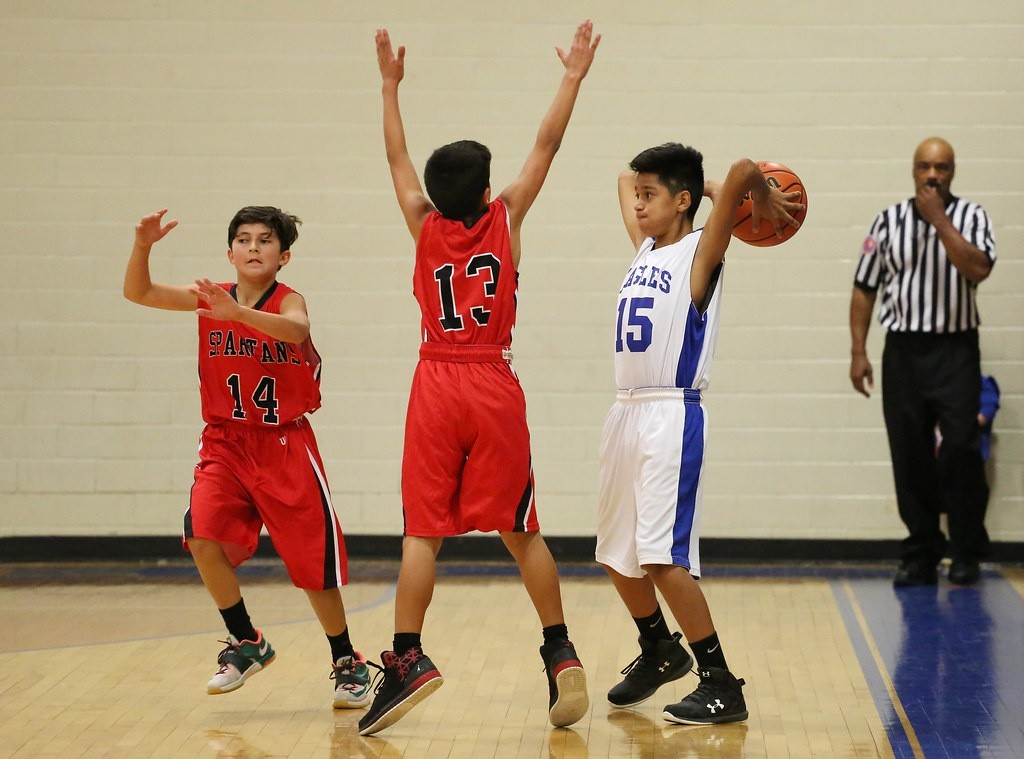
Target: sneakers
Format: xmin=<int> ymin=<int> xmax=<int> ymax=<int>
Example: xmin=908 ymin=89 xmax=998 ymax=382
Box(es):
xmin=329 ymin=652 xmax=372 ymax=709
xmin=539 ymin=640 xmax=590 ymax=727
xmin=358 ymin=646 xmax=444 ymax=735
xmin=608 ymin=631 xmax=749 ymax=725
xmin=207 ymin=629 xmax=276 ymax=695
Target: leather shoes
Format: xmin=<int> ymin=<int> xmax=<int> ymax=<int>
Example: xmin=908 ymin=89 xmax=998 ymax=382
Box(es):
xmin=893 ymin=545 xmax=982 ymax=586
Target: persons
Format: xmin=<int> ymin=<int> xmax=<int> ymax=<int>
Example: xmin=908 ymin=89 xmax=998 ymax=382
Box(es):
xmin=123 ymin=205 xmax=374 ymax=709
xmin=355 ymin=19 xmax=603 ymax=735
xmin=592 ymin=140 xmax=807 ymax=726
xmin=849 ymin=136 xmax=998 ymax=589
xmin=932 ymin=374 xmax=1008 ymax=579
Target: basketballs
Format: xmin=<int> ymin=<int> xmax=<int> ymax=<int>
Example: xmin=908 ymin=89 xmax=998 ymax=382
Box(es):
xmin=724 ymin=159 xmax=809 ymax=248
